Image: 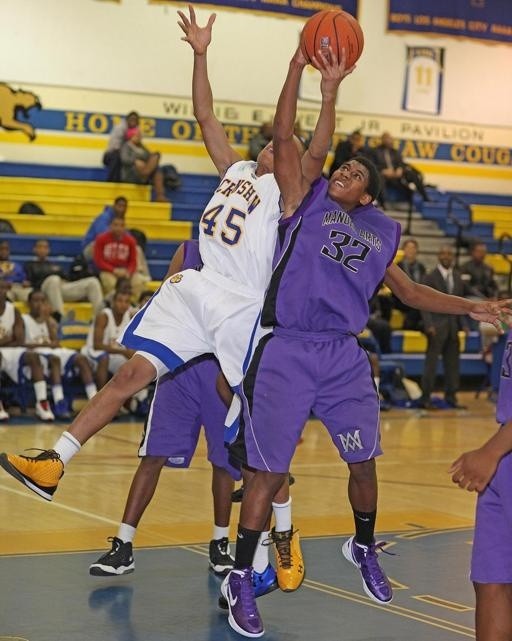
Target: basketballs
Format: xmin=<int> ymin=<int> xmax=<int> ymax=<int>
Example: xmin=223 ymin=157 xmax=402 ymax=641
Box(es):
xmin=300 ymin=10 xmax=363 ymax=69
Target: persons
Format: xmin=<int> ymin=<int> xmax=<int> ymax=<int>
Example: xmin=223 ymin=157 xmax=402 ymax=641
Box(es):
xmin=446 ymin=309 xmax=512 ymax=641
xmin=249 ymin=119 xmax=511 ymax=411
xmin=86 ymin=233 xmax=245 ymax=577
xmin=1 ymin=109 xmax=169 ymax=419
xmin=217 ymin=30 xmax=510 ymax=639
xmin=1 ymin=5 xmax=357 ymax=596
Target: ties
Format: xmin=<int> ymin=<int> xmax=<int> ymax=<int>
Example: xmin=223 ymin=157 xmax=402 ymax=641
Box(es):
xmin=446 ymin=273 xmax=453 ymax=294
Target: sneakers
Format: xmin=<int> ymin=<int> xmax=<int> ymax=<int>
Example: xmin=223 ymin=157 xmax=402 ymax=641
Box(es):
xmin=341 ymin=529 xmax=398 ymax=608
xmin=119 ymin=399 xmax=150 ymax=418
xmin=217 ymin=564 xmax=284 ymax=610
xmin=35 ymin=399 xmax=72 ymax=423
xmin=207 ymin=535 xmax=236 ymax=575
xmin=262 ymin=525 xmax=307 ymax=598
xmin=220 ymin=567 xmax=268 ymax=640
xmin=0 ymin=447 xmax=66 ymax=502
xmin=89 ymin=535 xmax=139 ymax=578
xmin=0 ymin=401 xmax=11 ymax=421
xmin=379 ymin=402 xmax=392 ymax=413
